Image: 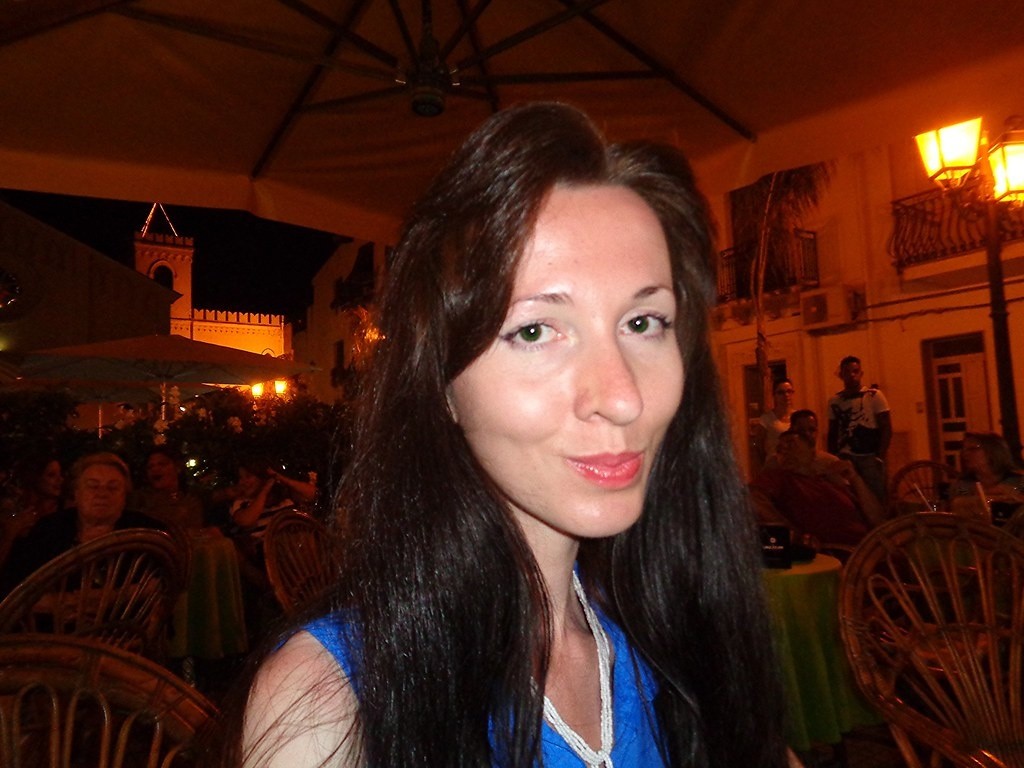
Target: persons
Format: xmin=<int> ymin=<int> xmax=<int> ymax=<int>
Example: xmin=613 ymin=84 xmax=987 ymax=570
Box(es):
xmin=227 ymin=102 xmax=784 ymax=768
xmin=0 ymin=357 xmax=1024 ymax=660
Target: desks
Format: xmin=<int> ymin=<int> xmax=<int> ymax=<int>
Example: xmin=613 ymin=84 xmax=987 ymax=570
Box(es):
xmin=752 ymin=553 xmax=883 ymax=768
xmin=149 ymin=533 xmax=250 ymax=689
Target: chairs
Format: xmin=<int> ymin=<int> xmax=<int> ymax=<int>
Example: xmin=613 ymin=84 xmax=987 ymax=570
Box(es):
xmin=892 ymin=459 xmax=961 ymax=503
xmin=0 ymin=632 xmax=225 ymax=768
xmin=0 ymin=528 xmax=184 ymax=654
xmin=263 ymin=509 xmax=340 ymax=611
xmin=838 ymin=511 xmax=1024 ymax=768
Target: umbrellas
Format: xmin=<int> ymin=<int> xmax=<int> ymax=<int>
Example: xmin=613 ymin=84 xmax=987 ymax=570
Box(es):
xmin=17 ymin=336 xmax=323 ymax=416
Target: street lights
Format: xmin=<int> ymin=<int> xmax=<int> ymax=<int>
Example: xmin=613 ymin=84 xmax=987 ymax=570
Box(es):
xmin=916 ymin=115 xmax=1023 ymax=467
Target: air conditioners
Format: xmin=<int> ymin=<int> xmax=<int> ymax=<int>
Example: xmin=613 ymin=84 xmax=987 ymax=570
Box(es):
xmin=799 ymin=285 xmax=855 ymax=331
